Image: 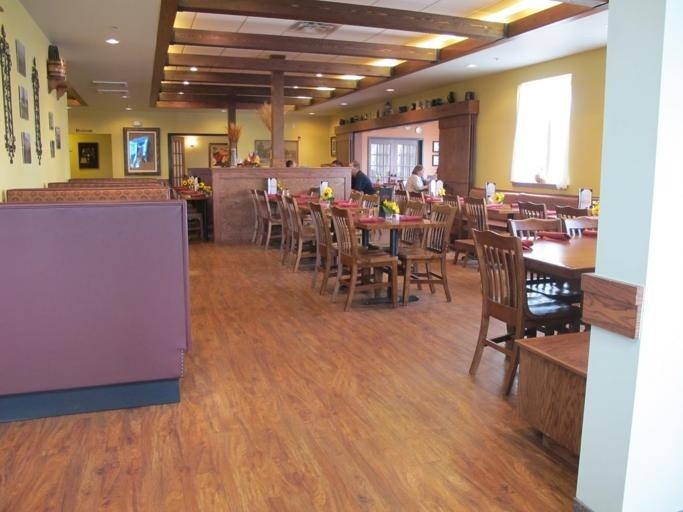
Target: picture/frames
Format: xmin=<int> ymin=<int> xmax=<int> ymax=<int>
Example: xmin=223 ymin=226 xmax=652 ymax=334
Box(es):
xmin=78 ymin=141 xmax=99 ymax=169
xmin=209 ymin=142 xmax=229 ymax=167
xmin=256 ymin=139 xmax=299 ymax=167
xmin=433 ymin=154 xmax=440 ymax=166
xmin=123 ymin=127 xmax=161 ymax=176
xmin=433 ymin=140 xmax=440 ymax=153
xmin=332 ymin=136 xmax=338 ymax=157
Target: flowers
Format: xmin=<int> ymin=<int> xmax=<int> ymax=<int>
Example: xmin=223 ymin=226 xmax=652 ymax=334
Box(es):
xmin=321 ymin=188 xmax=333 ymax=200
xmin=225 ymin=123 xmax=241 ymax=168
xmin=382 ymin=199 xmax=399 ymax=213
xmin=247 ymin=152 xmax=260 ymax=164
xmin=438 ymin=188 xmax=445 ymax=196
xmin=492 ymin=192 xmax=504 ymax=203
xmin=591 ymin=202 xmax=599 ymax=216
xmin=183 ymin=176 xmax=211 ymax=193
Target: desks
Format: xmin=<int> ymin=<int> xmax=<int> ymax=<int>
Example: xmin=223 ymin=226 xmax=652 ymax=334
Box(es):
xmin=454 ymin=234 xmax=597 ymax=332
xmin=461 ymin=203 xmax=557 ymax=231
xmin=350 ymin=213 xmax=449 ymax=305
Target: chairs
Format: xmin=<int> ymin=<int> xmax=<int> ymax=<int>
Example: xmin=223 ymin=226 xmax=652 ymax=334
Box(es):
xmin=355 ymin=193 xmax=379 ymax=244
xmin=367 ymin=201 xmax=427 ymax=290
xmin=380 ymin=202 xmax=457 ymax=308
xmin=283 ymin=195 xmax=324 ymax=272
xmin=275 ymin=193 xmax=295 ymax=264
xmin=463 ymin=196 xmax=488 ymax=230
xmin=506 ymin=218 xmax=582 ymax=304
xmin=250 ymin=188 xmax=261 ymax=245
xmin=408 ymin=191 xmax=425 ymax=204
xmin=308 ymin=202 xmax=379 ymax=296
xmin=517 ymin=200 xmax=546 ymax=219
xmin=469 ymin=226 xmax=581 ymax=396
xmin=351 ymin=190 xmax=365 ymax=201
xmin=255 ymin=189 xmax=282 ymax=250
xmin=442 ymin=194 xmax=467 ymax=240
xmin=553 ymin=203 xmax=591 ymax=218
xmin=563 ymin=217 xmax=597 ymax=237
xmin=170 ymin=188 xmax=204 ymax=241
xmin=329 ymin=206 xmax=399 ymax=312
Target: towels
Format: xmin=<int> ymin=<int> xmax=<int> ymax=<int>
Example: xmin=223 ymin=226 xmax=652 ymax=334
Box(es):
xmin=511 ymin=203 xmax=519 ymax=208
xmin=536 ymin=231 xmax=571 ymax=241
xmin=297 ymin=200 xmax=307 ymax=204
xmin=547 ymin=210 xmax=556 ymax=215
xmin=339 ymin=202 xmax=358 ymax=207
xmin=182 ymin=191 xmax=197 ymax=194
xmin=521 ymin=240 xmax=534 ymax=250
xmin=267 ymin=195 xmax=276 ymax=199
xmin=300 ymin=194 xmax=317 ymax=199
xmin=400 ymin=215 xmax=423 ymax=222
xmin=191 ymin=194 xmax=206 ymax=197
xmin=359 ymin=217 xmax=385 ymax=223
xmin=176 ymin=188 xmax=185 ymax=191
xmin=431 ymin=197 xmax=441 ymax=201
xmin=334 ymin=201 xmax=350 ymax=204
xmin=582 ymin=229 xmax=598 ymax=237
xmin=487 ymin=203 xmax=504 ymax=208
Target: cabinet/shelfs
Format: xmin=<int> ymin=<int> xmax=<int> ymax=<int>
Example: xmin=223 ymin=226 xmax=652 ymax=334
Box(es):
xmin=513 ymin=329 xmax=591 ymax=465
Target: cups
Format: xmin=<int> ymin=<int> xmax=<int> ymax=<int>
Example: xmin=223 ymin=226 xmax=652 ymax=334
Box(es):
xmin=385 ymin=212 xmax=391 ymax=223
xmin=281 ymin=188 xmax=290 ymax=198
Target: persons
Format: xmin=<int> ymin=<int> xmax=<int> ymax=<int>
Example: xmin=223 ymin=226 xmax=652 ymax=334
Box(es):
xmin=405 ymin=165 xmax=429 ymax=192
xmin=349 ymin=160 xmax=376 ymax=195
xmin=286 ymin=160 xmax=297 ymax=167
xmin=330 ymin=159 xmax=345 ymax=168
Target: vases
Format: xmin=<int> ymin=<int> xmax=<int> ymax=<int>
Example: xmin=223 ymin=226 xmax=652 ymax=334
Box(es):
xmin=384 ymin=212 xmax=392 ymax=220
